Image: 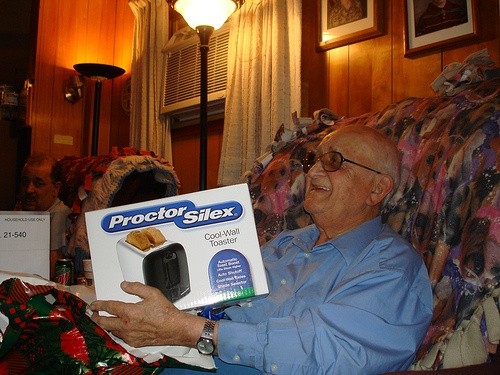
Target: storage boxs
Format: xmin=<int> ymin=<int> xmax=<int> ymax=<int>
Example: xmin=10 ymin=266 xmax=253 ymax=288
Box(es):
xmin=0 ymin=211 xmax=50 ymax=281
xmin=86 ymin=184 xmax=269 ymax=316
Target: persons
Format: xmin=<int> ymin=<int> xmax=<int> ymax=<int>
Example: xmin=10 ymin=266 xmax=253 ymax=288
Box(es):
xmin=327 ymin=0 xmax=369 ymax=31
xmin=15 ymin=153 xmax=71 ymax=281
xmin=412 ymin=0 xmax=469 ymax=37
xmin=89 ymin=124 xmax=434 ymax=375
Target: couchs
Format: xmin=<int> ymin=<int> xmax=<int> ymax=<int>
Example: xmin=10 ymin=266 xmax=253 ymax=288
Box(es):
xmin=245 ymin=48 xmax=500 ymax=375
xmin=57 ymin=148 xmax=178 ymax=260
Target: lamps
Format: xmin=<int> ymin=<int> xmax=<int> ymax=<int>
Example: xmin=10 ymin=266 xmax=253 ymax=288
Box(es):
xmin=73 ymin=63 xmax=126 ymax=156
xmin=167 ymin=0 xmax=245 ymax=190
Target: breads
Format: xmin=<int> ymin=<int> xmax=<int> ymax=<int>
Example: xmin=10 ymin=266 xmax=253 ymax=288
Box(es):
xmin=126 ymin=227 xmax=166 ymax=251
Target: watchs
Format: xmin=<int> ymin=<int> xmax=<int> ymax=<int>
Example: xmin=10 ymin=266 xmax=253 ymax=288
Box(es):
xmin=197 ymin=318 xmax=216 ymax=355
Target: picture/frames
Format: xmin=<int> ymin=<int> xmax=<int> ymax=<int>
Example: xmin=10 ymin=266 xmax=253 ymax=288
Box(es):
xmin=316 ymin=0 xmax=383 ymax=52
xmin=402 ymin=0 xmax=482 ymax=58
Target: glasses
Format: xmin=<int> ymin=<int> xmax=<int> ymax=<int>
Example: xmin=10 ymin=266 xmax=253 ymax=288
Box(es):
xmin=303 ymin=151 xmax=382 ymax=175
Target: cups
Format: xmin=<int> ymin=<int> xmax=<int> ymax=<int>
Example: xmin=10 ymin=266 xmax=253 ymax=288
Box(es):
xmin=82 ymin=259 xmax=95 ymax=289
